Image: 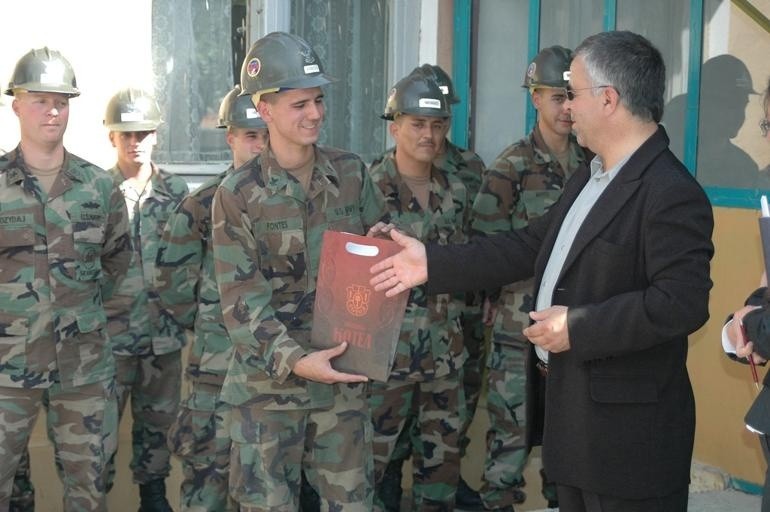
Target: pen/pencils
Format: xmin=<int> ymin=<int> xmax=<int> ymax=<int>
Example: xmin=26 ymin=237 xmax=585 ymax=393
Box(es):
xmin=738 ymin=318 xmax=761 ymax=391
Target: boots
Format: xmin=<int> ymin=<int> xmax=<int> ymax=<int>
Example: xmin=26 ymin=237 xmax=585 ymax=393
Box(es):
xmin=138 ymin=479 xmax=174 ymax=512
xmin=298 ymin=470 xmax=321 ymax=512
xmin=380 ymin=460 xmax=404 ymax=511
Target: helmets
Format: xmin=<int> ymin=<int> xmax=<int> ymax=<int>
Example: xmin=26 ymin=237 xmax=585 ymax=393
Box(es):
xmin=521 ymin=45 xmax=573 ymax=90
xmin=224 ymin=95 xmax=267 ymax=130
xmin=4 ymin=46 xmax=81 ymax=98
xmin=104 ymin=88 xmax=165 ymax=133
xmin=235 ymin=31 xmax=338 ymax=108
xmin=215 ymin=84 xmax=240 ymax=130
xmin=701 ymin=53 xmax=762 ymax=97
xmin=410 ymin=63 xmax=460 ymax=104
xmin=379 ymin=73 xmax=451 ymax=120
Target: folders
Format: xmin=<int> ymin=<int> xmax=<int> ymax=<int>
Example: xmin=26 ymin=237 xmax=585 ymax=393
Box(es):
xmin=757 ymin=194 xmax=770 ymax=290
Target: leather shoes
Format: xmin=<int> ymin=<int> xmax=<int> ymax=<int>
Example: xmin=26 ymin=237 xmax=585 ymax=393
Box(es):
xmin=457 ymin=473 xmax=482 ymax=506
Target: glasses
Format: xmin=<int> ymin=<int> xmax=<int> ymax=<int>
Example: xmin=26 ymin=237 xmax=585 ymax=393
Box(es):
xmin=565 ymin=84 xmax=606 ymax=101
xmin=757 ymin=118 xmax=770 ymax=137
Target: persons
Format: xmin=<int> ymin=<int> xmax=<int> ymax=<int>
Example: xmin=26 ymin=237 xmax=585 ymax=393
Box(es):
xmin=0 ymin=47 xmax=133 ymax=512
xmin=369 ymin=76 xmax=467 ymax=509
xmin=377 ymin=64 xmax=487 ymax=511
xmin=469 ymin=46 xmax=596 ymax=511
xmin=721 ymin=76 xmax=770 ymax=511
xmin=102 ymin=89 xmax=190 ymax=512
xmin=697 ymin=54 xmax=761 ymax=188
xmin=370 ymin=30 xmax=715 ymax=512
xmin=211 ymin=32 xmax=417 ymax=511
xmin=153 ymin=86 xmax=269 ymax=512
